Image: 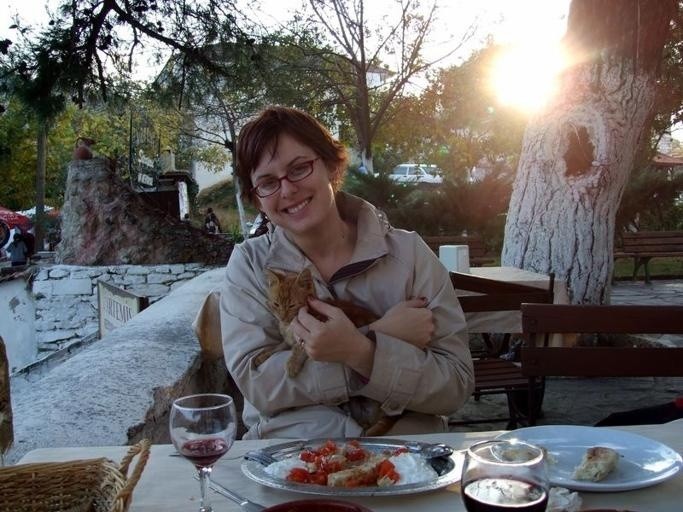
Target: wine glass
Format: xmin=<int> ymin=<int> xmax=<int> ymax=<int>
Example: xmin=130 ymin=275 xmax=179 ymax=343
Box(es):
xmin=166 ymin=394 xmax=238 ymax=512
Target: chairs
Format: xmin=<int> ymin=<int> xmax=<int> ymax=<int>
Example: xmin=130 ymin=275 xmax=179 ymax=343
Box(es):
xmin=440 ymin=271 xmax=555 ymax=429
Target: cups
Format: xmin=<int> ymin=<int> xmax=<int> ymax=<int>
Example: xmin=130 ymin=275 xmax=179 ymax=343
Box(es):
xmin=463 ymin=435 xmax=550 ymax=512
xmin=261 ymin=499 xmax=375 ymax=512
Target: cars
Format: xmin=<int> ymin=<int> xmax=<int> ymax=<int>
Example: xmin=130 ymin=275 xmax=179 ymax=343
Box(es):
xmin=374 ymin=163 xmax=474 ymax=189
xmin=246 ymin=212 xmax=269 ymax=238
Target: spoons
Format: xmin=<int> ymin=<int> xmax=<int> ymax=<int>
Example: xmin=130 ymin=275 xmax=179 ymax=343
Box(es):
xmin=292 ymin=442 xmax=456 ymax=465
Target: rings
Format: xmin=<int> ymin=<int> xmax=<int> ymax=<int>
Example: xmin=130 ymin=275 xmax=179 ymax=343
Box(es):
xmin=298 ymin=339 xmax=304 ymax=348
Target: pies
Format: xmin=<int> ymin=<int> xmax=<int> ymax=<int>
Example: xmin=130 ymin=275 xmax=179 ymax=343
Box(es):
xmin=569 ymin=447 xmax=618 ymax=481
xmin=500 ymin=444 xmax=557 ymax=464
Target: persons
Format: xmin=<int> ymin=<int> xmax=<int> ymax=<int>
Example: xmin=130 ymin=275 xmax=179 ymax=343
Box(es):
xmin=6 ymin=234 xmax=27 ymax=266
xmin=218 ymin=105 xmax=473 ymax=439
xmin=203 ymin=206 xmax=221 ymax=234
xmin=182 ymin=214 xmax=192 ymax=224
xmin=204 ymin=213 xmax=220 ymax=235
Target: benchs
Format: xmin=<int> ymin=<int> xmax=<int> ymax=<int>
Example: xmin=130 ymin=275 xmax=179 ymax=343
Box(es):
xmin=519 ymin=304 xmax=683 ymax=425
xmin=420 ymin=234 xmax=496 ymax=266
xmin=614 ymin=230 xmax=682 ymax=284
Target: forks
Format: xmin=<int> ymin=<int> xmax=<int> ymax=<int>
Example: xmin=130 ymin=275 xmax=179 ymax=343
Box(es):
xmin=166 ymin=450 xmax=278 ymax=466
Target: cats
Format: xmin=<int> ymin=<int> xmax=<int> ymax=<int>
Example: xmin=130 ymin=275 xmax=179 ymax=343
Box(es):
xmin=251 ymin=267 xmax=399 ymax=437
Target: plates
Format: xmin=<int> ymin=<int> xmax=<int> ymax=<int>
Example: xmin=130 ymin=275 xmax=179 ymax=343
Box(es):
xmin=236 ymin=436 xmax=466 ymax=497
xmin=488 ymin=422 xmax=683 ymax=493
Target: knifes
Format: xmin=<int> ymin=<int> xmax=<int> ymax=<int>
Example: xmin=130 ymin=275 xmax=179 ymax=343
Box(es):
xmin=193 ymin=471 xmax=266 ymax=511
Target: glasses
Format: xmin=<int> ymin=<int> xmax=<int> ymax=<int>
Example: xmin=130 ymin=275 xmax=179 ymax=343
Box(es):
xmin=251 ymin=155 xmax=320 ymax=199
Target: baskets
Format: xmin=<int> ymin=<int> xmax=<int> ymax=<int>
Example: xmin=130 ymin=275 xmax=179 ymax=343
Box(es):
xmin=0 ymin=438 xmax=152 ymax=511
